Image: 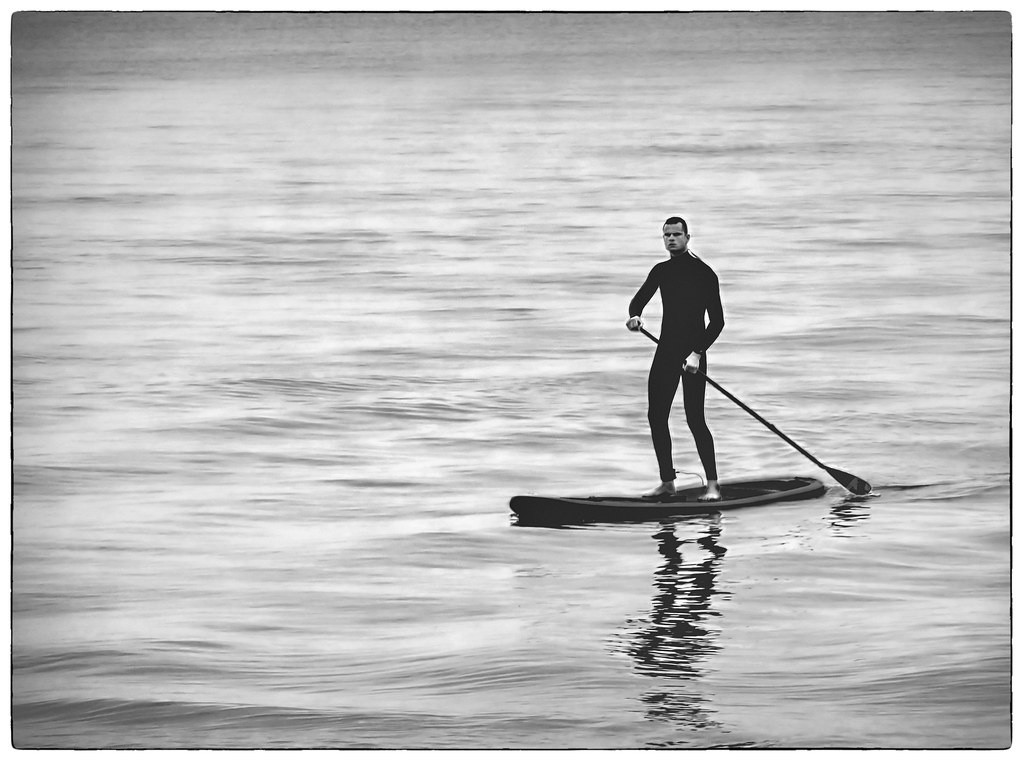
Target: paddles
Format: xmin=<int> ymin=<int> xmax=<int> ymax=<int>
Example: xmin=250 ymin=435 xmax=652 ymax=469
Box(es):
xmin=639 ymin=327 xmax=874 ymax=496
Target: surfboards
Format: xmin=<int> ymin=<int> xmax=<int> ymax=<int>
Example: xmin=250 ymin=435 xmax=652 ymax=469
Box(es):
xmin=508 ymin=476 xmax=823 ymax=525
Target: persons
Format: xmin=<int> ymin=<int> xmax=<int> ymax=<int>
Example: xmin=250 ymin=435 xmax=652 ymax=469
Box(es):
xmin=627 ymin=217 xmax=724 ymax=502
